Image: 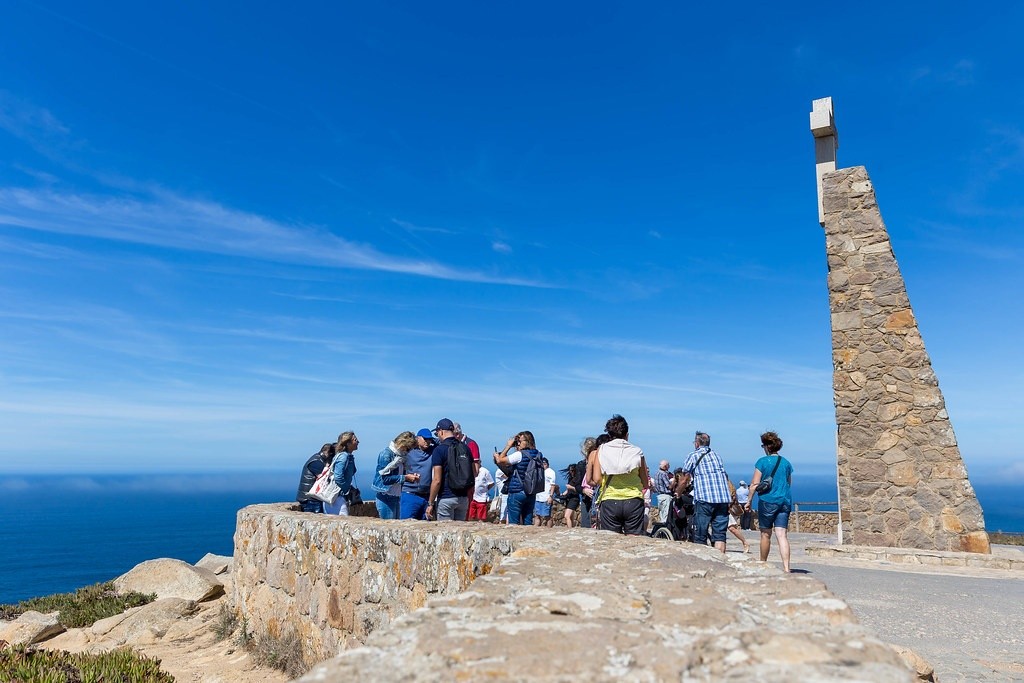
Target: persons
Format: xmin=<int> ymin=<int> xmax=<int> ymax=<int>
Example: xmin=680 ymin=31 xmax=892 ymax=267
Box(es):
xmin=533 ymin=458 xmax=555 ymax=528
xmin=493 ymin=431 xmax=543 ymax=525
xmin=425 ymin=418 xmax=476 ymax=521
xmin=398 ymin=428 xmax=437 ymax=520
xmin=469 ymin=467 xmax=494 ymax=521
xmin=643 ymin=460 xmax=751 ymax=553
xmin=323 ymin=432 xmax=359 ymax=516
xmin=561 ymin=464 xmax=580 ymax=528
xmin=744 ymin=432 xmax=794 ymax=573
xmin=576 ymin=434 xmax=611 ymax=529
xmin=593 ymin=414 xmax=649 ymax=535
xmin=674 ymin=432 xmax=729 ymax=553
xmin=372 ymin=431 xmax=420 ymax=518
xmin=296 ymin=444 xmax=334 ymax=513
xmin=453 ymin=423 xmax=480 ymax=520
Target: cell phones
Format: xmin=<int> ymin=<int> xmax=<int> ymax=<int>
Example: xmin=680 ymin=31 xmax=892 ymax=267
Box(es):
xmin=512 ymin=440 xmax=519 ymax=446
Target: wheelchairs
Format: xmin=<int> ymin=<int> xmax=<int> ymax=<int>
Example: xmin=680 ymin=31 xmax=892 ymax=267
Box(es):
xmin=652 ymin=495 xmax=714 ymax=547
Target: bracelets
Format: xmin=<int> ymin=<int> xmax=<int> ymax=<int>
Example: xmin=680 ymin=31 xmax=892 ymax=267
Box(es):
xmin=550 ymin=495 xmax=554 ymax=498
xmin=428 ymin=501 xmax=434 ymax=505
xmin=748 ymin=502 xmax=750 ymax=503
xmin=507 ymin=445 xmax=510 ymax=446
xmin=562 ymin=493 xmax=563 ymax=496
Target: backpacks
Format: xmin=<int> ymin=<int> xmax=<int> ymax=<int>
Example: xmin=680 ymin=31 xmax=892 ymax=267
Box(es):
xmin=441 ymin=438 xmax=475 ymax=493
xmin=515 ymin=450 xmax=545 ymax=495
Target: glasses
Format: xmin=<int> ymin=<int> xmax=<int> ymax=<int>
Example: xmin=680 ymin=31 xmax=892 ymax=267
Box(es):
xmin=693 ymin=441 xmax=697 ymax=444
xmin=569 ymin=470 xmax=574 ymax=472
xmin=761 ymin=444 xmax=766 ymax=448
xmin=434 ymin=429 xmax=440 ymax=437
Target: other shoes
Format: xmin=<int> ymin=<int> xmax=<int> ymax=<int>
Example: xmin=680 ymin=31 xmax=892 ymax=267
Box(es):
xmin=742 ymin=528 xmax=750 ymax=532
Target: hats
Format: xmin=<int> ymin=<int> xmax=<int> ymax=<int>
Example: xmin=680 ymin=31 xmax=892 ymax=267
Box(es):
xmin=417 ymin=429 xmax=434 ymax=439
xmin=431 ymin=418 xmax=454 ymax=431
xmin=739 ymin=481 xmax=746 ymax=485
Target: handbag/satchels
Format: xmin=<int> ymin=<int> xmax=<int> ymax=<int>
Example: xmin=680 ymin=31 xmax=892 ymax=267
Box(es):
xmin=306 ymin=453 xmax=349 ymax=505
xmin=756 ymin=477 xmax=772 ymax=495
xmin=351 ymin=488 xmax=364 ymax=505
xmin=730 ymin=503 xmax=744 ymax=516
